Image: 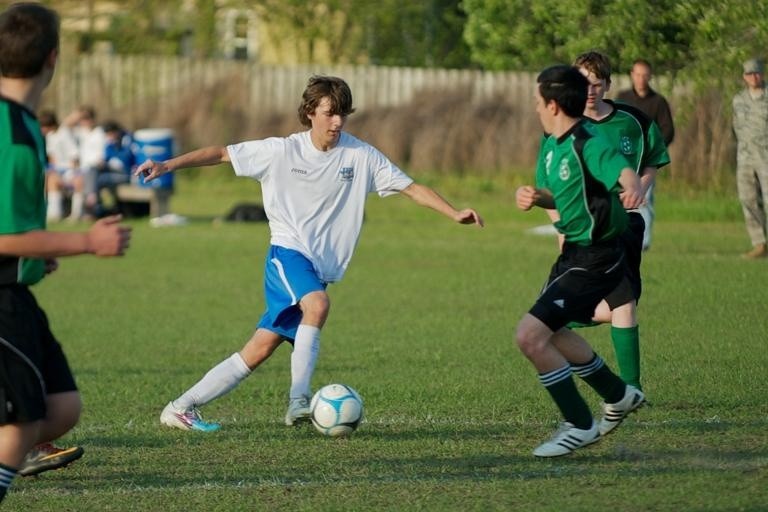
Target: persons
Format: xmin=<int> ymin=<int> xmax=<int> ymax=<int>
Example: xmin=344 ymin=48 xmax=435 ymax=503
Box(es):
xmin=133 ymin=75 xmax=485 ymax=433
xmin=536 ymin=51 xmax=671 ymax=393
xmin=732 ymin=59 xmax=768 ymax=259
xmin=514 ymin=66 xmax=647 ymax=459
xmin=615 ymin=60 xmax=676 ymax=252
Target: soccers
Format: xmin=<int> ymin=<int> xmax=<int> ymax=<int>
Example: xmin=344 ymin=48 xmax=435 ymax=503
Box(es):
xmin=310 ymin=384 xmax=363 ymax=437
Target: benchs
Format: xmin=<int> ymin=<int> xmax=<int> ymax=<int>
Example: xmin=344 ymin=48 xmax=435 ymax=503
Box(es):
xmin=59 ymin=181 xmax=168 ymax=223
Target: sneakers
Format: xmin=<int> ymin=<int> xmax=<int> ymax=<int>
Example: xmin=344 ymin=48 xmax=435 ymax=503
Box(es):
xmin=742 ymin=243 xmax=766 ymax=260
xmin=599 ymin=384 xmax=645 ymax=437
xmin=16 ymin=442 xmax=84 ymax=477
xmin=282 ymin=396 xmax=316 ymax=427
xmin=532 ymin=415 xmax=600 ymax=458
xmin=158 ymin=400 xmax=221 ymax=434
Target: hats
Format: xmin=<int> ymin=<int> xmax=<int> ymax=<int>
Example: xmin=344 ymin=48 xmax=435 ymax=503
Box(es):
xmin=742 ymin=57 xmax=762 ymax=74
